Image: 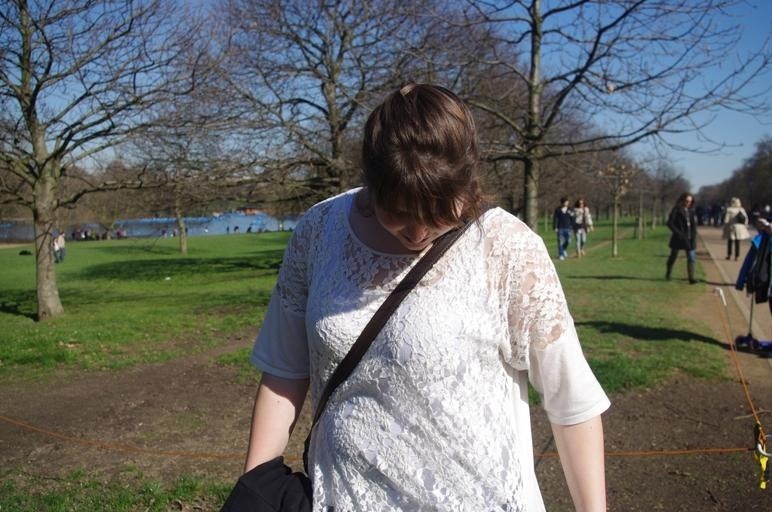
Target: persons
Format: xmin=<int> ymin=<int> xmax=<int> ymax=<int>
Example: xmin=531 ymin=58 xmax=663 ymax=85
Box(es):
xmin=569 ymin=197 xmax=594 ymax=258
xmin=552 ymin=195 xmax=571 ymax=261
xmin=721 ymin=196 xmax=747 ymax=263
xmin=754 ymin=216 xmax=772 ymax=358
xmin=693 ymin=200 xmax=772 ymax=227
xmin=242 ymin=74 xmax=608 ymax=512
xmin=661 ymin=190 xmax=700 ymax=287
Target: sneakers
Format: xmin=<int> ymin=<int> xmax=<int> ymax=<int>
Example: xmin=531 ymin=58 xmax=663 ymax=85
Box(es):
xmin=559 ymin=250 xmax=585 ymax=261
xmin=726 ymin=255 xmax=739 ymax=260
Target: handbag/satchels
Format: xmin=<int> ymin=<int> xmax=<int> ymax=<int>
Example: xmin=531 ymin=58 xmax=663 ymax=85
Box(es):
xmin=737 ymin=211 xmax=746 ymax=224
